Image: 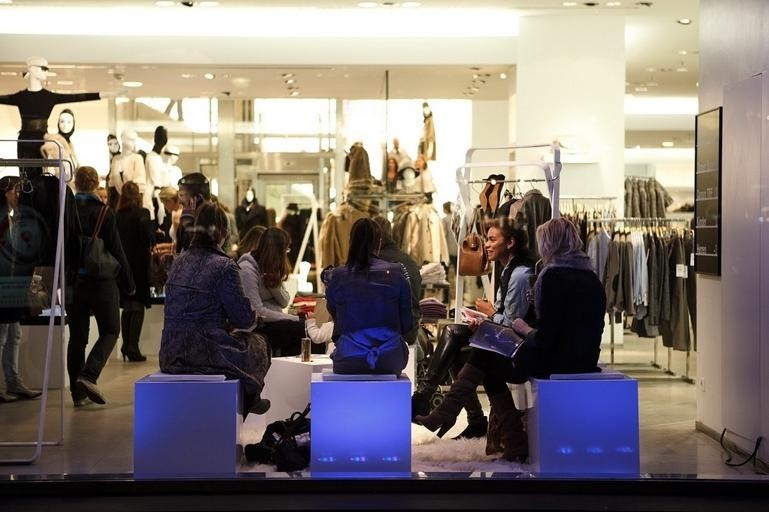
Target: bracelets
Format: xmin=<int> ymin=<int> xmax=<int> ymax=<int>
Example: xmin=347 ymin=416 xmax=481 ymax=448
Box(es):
xmin=491 ymin=311 xmax=497 ymax=321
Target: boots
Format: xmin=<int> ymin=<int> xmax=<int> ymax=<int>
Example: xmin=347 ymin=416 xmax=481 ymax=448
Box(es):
xmin=121 ymin=310 xmax=146 ymax=361
xmin=411 ymin=362 xmax=528 ymax=465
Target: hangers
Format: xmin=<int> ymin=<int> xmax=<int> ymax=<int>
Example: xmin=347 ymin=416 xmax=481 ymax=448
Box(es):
xmin=558 ymin=174 xmax=696 ymax=240
xmin=467 ymin=179 xmax=543 ymax=205
xmin=336 ymin=191 xmax=382 ymax=213
xmin=389 ymin=198 xmax=436 ymax=222
xmin=0 ymin=166 xmax=57 ymax=189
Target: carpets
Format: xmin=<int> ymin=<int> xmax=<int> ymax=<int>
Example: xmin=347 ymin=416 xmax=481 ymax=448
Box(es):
xmin=235 ymin=384 xmax=530 ymax=476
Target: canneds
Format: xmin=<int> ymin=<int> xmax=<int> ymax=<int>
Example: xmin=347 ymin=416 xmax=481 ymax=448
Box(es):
xmin=301 ymin=338 xmax=311 ymax=362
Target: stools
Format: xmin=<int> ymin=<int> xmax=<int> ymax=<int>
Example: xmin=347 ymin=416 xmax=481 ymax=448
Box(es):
xmin=133 ymin=368 xmax=246 ymax=476
xmin=519 ymin=368 xmax=640 ymax=472
xmin=309 ymin=370 xmax=411 ymax=472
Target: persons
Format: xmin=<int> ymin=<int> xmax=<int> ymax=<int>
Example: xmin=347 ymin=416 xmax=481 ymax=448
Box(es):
xmin=159 ymin=173 xmax=338 ymax=415
xmin=0 ymin=166 xmax=156 ymax=406
xmin=1 ymin=55 xmax=183 ymax=225
xmin=382 ymin=137 xmax=435 ymax=206
xmin=323 ymin=217 xmax=412 ymax=375
xmin=415 ymin=216 xmax=607 ymax=464
xmin=411 ymin=218 xmax=545 ymax=441
xmin=371 ymin=215 xmax=421 ymax=345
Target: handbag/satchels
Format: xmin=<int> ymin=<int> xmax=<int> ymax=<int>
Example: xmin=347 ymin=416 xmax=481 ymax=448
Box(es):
xmin=83 ymin=205 xmax=122 ymax=279
xmin=141 ymin=238 xmax=168 ymax=286
xmin=485 ymin=407 xmax=535 ymax=455
xmin=245 ymin=403 xmax=310 ymax=472
xmin=459 ymin=207 xmax=492 ymax=276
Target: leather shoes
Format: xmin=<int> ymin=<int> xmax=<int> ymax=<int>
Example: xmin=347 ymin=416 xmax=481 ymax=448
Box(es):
xmin=245 ymin=394 xmax=271 ymax=414
xmin=0 ymin=385 xmax=41 ymax=402
xmin=72 ymin=376 xmax=106 ymax=406
